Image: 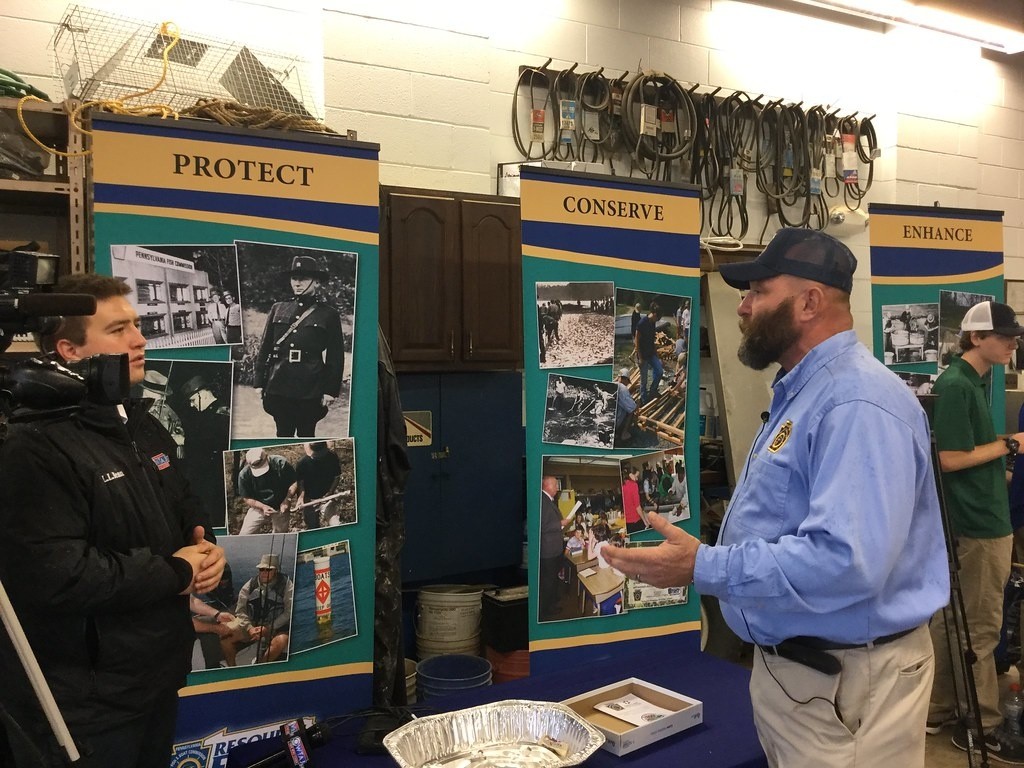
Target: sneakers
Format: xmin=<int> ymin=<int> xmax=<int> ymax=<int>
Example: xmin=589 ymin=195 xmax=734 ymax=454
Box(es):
xmin=952 ymin=725 xmax=1024 ymax=766
xmin=924 ymin=711 xmax=959 ymax=737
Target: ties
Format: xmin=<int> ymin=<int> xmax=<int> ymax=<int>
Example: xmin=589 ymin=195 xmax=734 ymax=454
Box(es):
xmin=225 ymin=304 xmax=232 ymax=326
xmin=215 ymin=303 xmax=222 ymax=321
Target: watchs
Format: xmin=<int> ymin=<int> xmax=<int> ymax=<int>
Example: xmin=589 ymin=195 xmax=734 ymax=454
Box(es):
xmin=1003 ymin=436 xmax=1020 ymax=456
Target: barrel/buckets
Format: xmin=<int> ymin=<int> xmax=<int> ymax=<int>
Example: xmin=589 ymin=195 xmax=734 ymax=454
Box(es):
xmin=414 ymin=653 xmax=495 ymax=705
xmin=486 ymin=645 xmax=532 ymax=686
xmin=412 ymin=584 xmax=482 ymax=667
xmin=403 ymin=658 xmax=419 ymax=705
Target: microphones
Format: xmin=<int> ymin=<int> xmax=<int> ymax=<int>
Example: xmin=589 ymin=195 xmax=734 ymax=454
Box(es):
xmin=761 ymin=411 xmax=770 ymax=422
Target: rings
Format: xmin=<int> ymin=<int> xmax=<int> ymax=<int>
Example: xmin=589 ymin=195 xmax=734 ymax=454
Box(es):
xmin=636 ymin=574 xmax=641 ymax=582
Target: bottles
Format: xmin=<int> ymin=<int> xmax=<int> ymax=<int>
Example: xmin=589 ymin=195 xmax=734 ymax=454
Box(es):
xmin=1003 ymin=683 xmax=1024 ymax=741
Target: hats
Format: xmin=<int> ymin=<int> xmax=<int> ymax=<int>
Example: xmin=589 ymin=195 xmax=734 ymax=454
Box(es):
xmin=928 ymin=313 xmax=935 ymax=323
xmin=180 ymin=374 xmax=210 ymax=398
xmin=288 ymin=253 xmax=328 ymax=278
xmin=717 ymin=224 xmax=861 ymax=299
xmin=254 ymin=553 xmax=281 ymax=571
xmin=963 ymin=301 xmax=1024 ymax=339
xmin=303 ymin=441 xmax=328 ymax=459
xmin=222 ymin=291 xmax=233 ymax=299
xmin=619 ymin=368 xmax=633 ymax=383
xmin=207 ymin=290 xmax=220 ymax=299
xmin=246 ymin=450 xmax=271 ymax=478
xmin=135 ymin=369 xmax=173 ymax=397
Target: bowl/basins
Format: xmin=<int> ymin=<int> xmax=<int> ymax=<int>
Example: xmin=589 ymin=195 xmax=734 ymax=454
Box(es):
xmin=382 ymin=699 xmax=606 ymax=768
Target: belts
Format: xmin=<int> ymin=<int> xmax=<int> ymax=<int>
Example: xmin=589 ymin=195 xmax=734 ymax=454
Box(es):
xmin=750 ymin=629 xmax=943 ymax=676
xmin=273 ymin=349 xmax=330 ymax=365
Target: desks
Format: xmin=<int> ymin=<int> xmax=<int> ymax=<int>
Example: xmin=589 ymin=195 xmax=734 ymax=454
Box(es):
xmin=225 ymin=644 xmax=769 ymax=768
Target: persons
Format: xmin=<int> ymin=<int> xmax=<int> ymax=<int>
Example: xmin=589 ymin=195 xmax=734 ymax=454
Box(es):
xmin=0 ymin=275 xmax=228 ymax=768
xmin=253 ymin=256 xmax=344 ymax=438
xmin=141 ymin=371 xmax=229 ymax=527
xmin=238 ymin=441 xmax=342 ymax=536
xmin=925 ymin=301 xmax=1024 ymax=765
xmin=189 ymin=554 xmax=293 ymax=669
xmin=538 ymin=294 xmax=686 ymax=621
xmin=206 ymin=290 xmax=242 ymax=344
xmin=600 ymin=225 xmax=952 ymax=768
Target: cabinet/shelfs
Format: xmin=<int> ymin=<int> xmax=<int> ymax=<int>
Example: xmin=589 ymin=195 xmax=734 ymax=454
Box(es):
xmin=381 ymin=185 xmax=525 ymax=371
xmin=0 ymin=96 xmax=87 ymax=356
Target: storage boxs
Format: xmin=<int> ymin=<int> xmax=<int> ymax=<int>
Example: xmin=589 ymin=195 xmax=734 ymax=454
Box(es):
xmin=559 ymin=675 xmax=704 ymax=758
xmin=381 ymin=698 xmax=607 ymax=767
